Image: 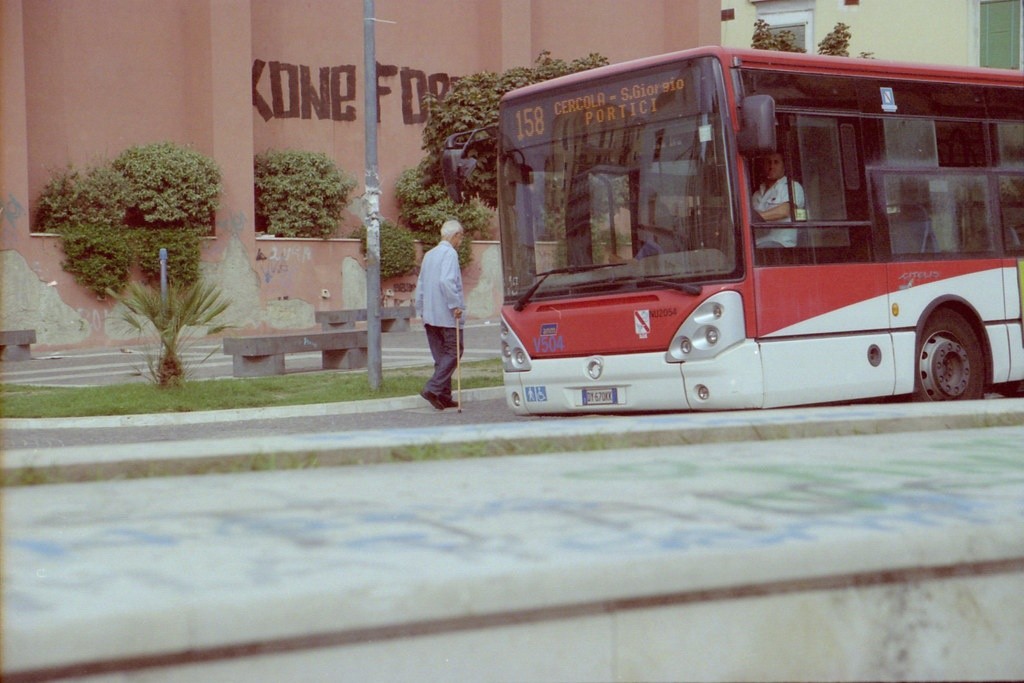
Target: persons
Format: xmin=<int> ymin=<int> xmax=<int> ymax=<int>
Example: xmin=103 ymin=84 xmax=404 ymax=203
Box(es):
xmin=413 ymin=219 xmax=466 ymax=411
xmin=751 ymin=151 xmax=806 ymax=248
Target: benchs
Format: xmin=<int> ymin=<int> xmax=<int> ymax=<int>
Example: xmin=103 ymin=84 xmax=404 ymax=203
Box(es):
xmin=315 ymin=304 xmax=413 ymax=337
xmin=0 ymin=329 xmax=36 ymax=360
xmin=222 ymin=328 xmax=369 ymax=375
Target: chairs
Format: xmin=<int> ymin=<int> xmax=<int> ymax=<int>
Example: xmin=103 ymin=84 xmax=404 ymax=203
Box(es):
xmin=891 ymin=204 xmax=930 ymax=254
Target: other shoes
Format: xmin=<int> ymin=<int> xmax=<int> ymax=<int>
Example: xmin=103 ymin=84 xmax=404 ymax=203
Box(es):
xmin=420 ymin=388 xmax=444 ymax=409
xmin=443 ymin=398 xmax=458 ymax=407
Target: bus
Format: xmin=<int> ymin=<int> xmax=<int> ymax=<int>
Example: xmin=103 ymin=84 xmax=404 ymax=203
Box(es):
xmin=438 ymin=45 xmax=1022 ymax=414
